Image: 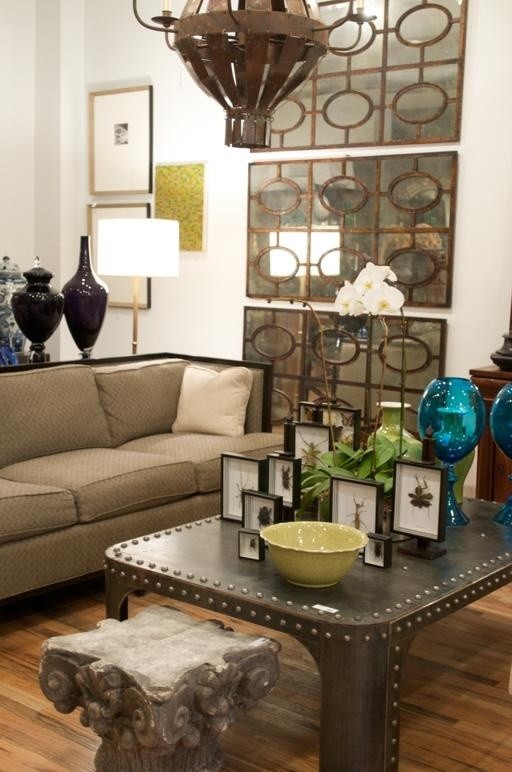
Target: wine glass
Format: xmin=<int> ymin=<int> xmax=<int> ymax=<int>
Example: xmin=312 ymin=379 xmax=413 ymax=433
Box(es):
xmin=490 ymin=383 xmax=512 ymax=526
xmin=413 ymin=375 xmax=489 ymax=529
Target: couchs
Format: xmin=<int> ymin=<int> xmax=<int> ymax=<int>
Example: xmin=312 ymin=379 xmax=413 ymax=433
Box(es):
xmin=1 ymin=348 xmax=286 ymax=606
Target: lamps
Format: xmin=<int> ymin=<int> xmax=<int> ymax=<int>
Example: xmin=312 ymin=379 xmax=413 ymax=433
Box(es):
xmin=263 ymin=225 xmax=342 ymax=425
xmin=94 ymin=217 xmax=180 ymax=353
xmin=132 ymin=1 xmax=378 ymax=148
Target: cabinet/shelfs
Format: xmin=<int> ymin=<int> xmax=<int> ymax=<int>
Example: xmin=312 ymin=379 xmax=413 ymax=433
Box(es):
xmin=469 ymin=361 xmax=512 ymax=504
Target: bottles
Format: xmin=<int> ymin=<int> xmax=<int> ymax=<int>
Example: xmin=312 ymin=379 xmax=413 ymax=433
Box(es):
xmin=1 ymin=234 xmax=107 ymax=368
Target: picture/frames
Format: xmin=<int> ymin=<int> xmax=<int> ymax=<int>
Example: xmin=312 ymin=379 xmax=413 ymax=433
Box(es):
xmin=218 ymin=395 xmax=451 ymax=570
xmin=87 ymin=84 xmax=153 ymax=195
xmin=83 ymin=201 xmax=150 ymax=310
xmin=152 ymin=159 xmax=208 ymax=252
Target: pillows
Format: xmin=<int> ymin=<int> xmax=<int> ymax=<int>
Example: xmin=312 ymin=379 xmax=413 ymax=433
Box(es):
xmin=171 ymin=360 xmax=255 ymax=441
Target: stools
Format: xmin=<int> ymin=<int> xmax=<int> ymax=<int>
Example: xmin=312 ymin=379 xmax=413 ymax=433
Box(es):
xmin=35 ymin=602 xmax=279 ymax=772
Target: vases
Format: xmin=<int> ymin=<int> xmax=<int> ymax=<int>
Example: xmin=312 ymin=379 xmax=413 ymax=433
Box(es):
xmin=367 ymin=399 xmax=424 ymax=464
xmin=62 ymin=235 xmax=108 ymax=357
xmin=429 ymin=407 xmax=474 ymax=504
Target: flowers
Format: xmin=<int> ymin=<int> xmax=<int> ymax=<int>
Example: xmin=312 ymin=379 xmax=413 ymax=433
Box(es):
xmin=292 ymin=256 xmax=409 ymax=537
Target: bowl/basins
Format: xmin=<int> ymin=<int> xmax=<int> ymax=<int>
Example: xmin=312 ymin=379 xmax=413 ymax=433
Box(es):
xmin=259 ymin=520 xmax=371 ymax=591
